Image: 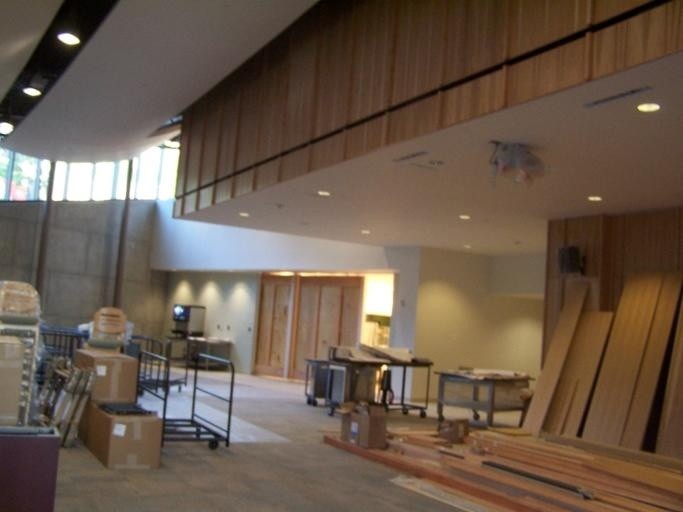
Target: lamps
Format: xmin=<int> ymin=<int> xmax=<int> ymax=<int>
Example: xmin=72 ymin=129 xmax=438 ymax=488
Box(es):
xmin=0 ymin=110 xmax=14 ymax=135
xmin=20 ymin=68 xmax=47 ymax=96
xmin=57 ymin=17 xmax=81 ymax=45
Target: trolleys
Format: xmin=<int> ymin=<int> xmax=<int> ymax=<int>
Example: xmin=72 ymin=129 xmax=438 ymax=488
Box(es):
xmin=137 ymin=351 xmax=234 ymax=449
xmin=131 ymin=337 xmax=190 ymax=398
xmin=33 ymin=326 xmax=88 ymax=386
xmin=300 ymin=343 xmax=432 ymax=418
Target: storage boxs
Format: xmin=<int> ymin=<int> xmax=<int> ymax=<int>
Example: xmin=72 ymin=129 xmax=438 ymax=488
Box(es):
xmin=73 ymin=348 xmax=139 ymax=402
xmin=334 ymin=400 xmax=387 ymax=448
xmin=186 ymin=338 xmax=232 ymax=367
xmin=74 ymin=397 xmax=162 ymax=472
xmin=0 ymin=314 xmax=37 ymax=428
